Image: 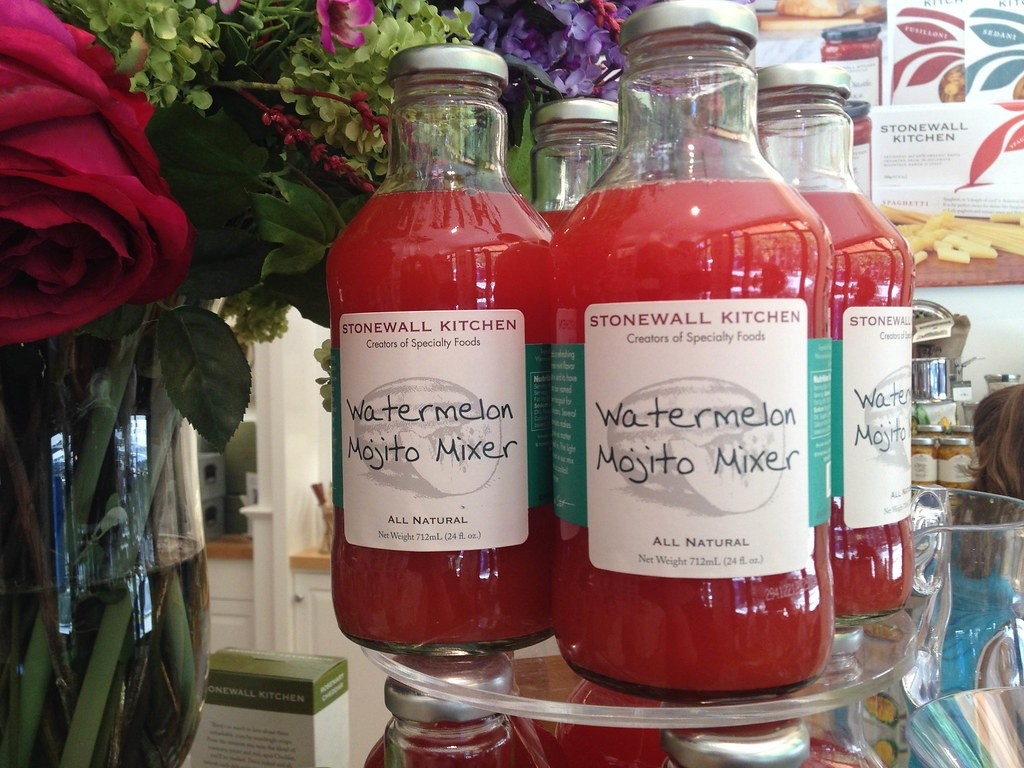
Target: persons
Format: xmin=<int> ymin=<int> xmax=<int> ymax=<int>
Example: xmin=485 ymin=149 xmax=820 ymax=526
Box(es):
xmin=905 ymin=386 xmax=1024 ymax=768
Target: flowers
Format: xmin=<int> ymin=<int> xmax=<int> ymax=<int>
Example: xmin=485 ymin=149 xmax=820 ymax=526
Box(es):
xmin=0 ymin=0 xmax=663 ymax=768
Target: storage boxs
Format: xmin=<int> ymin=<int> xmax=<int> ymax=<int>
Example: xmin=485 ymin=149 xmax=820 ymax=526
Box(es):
xmin=193 ymin=647 xmax=350 ymax=768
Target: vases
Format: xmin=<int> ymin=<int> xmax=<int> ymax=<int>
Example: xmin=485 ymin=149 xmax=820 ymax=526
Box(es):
xmin=0 ymin=299 xmax=211 ymax=768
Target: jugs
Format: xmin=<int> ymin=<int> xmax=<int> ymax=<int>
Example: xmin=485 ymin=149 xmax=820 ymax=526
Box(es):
xmin=904 ymin=487 xmax=1024 ymax=712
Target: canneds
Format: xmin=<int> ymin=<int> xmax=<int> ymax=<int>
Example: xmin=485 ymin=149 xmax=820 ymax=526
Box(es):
xmin=911 ymin=438 xmax=938 ymax=484
xmin=938 ymin=437 xmax=974 ymax=488
xmin=951 ymin=426 xmax=975 ymax=452
xmin=915 ymin=425 xmax=946 ymax=447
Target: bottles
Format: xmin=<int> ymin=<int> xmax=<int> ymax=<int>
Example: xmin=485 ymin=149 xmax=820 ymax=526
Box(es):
xmin=530 ymin=97 xmax=621 ymax=212
xmin=361 ymin=660 xmax=563 ymax=768
xmin=328 ymin=41 xmax=550 ymax=657
xmin=756 ymin=68 xmax=916 ymax=615
xmin=551 ymin=0 xmax=833 ymax=700
